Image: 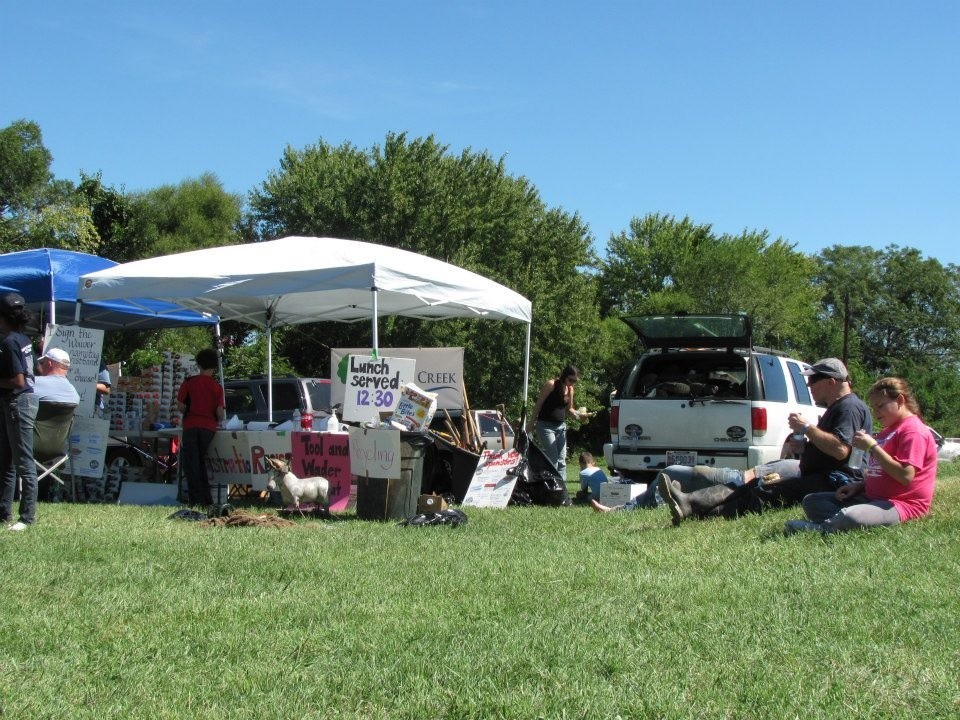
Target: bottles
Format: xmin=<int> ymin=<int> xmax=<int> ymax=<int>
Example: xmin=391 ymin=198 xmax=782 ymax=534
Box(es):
xmin=849 ymin=429 xmax=866 ymax=469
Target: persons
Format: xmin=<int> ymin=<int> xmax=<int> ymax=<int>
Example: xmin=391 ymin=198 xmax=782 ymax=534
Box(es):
xmin=591 ymin=457 xmax=801 ymax=511
xmin=0 ymin=292 xmax=42 ymax=531
xmin=95 ymin=359 xmax=111 ymax=419
xmin=177 ymin=349 xmax=224 ymax=508
xmin=34 ymin=347 xmax=81 ymax=403
xmin=657 ymin=358 xmax=872 ymax=523
xmin=525 ymin=365 xmax=587 ymax=484
xmin=783 ymin=377 xmax=937 ymax=532
xmin=577 ymin=452 xmax=608 ymax=502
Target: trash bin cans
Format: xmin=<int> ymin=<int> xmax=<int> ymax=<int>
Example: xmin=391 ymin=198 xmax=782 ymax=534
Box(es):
xmin=357 ymin=423 xmax=429 ymax=523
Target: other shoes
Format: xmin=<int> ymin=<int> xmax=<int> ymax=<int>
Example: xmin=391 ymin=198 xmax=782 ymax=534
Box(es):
xmin=784 ymin=519 xmax=822 ymax=535
xmin=8 ymin=522 xmax=27 ymax=531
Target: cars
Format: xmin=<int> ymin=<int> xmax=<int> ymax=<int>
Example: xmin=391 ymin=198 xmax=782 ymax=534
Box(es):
xmin=430 ymin=408 xmax=516 ymax=477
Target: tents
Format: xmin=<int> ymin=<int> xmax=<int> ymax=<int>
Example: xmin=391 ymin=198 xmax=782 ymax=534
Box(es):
xmin=75 ymin=236 xmax=531 ymax=420
xmin=0 ymin=248 xmax=227 ymax=419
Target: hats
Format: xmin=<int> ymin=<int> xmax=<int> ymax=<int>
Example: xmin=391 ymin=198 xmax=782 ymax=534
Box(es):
xmin=37 ymin=348 xmax=70 ymax=366
xmin=565 ymin=366 xmax=578 ymax=381
xmin=0 ymin=292 xmax=25 ymax=308
xmin=798 ymin=356 xmax=848 ymax=381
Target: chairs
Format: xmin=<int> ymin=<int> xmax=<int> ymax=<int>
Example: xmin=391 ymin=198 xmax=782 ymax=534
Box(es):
xmin=18 ymin=401 xmax=86 ymax=499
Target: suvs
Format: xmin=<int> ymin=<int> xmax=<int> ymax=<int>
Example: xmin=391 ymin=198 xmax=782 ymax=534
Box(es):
xmin=603 ymin=313 xmax=830 ymax=476
xmin=223 ymin=373 xmax=331 ymax=423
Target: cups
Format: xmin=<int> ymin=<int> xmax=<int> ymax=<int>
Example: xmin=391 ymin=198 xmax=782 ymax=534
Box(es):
xmin=292 ymin=409 xmax=301 ymax=431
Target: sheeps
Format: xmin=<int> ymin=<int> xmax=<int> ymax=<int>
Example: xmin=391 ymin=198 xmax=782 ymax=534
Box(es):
xmin=264 ymin=454 xmax=334 ymax=520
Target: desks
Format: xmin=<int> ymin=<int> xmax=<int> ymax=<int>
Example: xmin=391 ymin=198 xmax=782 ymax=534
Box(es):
xmin=103 ymin=428 xmax=176 ymax=471
xmin=161 ymin=427 xmax=349 ymax=519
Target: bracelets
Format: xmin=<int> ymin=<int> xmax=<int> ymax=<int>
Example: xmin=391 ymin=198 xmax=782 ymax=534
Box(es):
xmin=865 ymin=440 xmax=877 ymax=452
xmin=800 ymin=422 xmax=811 ymax=434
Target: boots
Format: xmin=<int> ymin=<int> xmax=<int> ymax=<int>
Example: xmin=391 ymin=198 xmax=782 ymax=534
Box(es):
xmin=657 ymin=473 xmax=735 ymax=521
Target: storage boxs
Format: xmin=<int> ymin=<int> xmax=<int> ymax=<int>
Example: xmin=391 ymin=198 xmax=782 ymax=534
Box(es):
xmin=600 ymin=481 xmax=648 ymax=509
